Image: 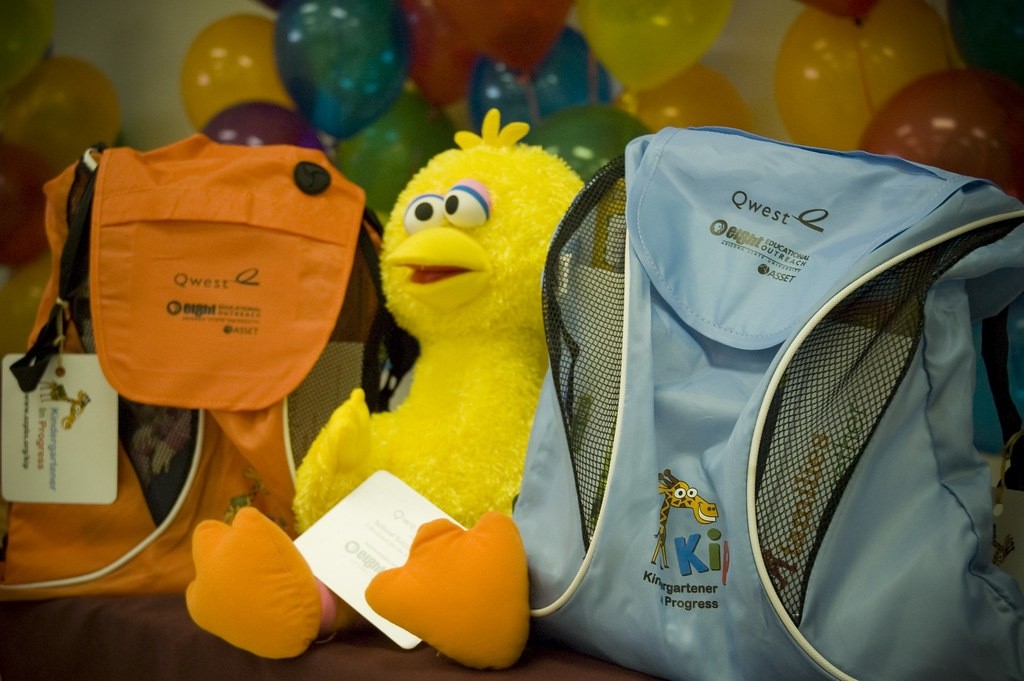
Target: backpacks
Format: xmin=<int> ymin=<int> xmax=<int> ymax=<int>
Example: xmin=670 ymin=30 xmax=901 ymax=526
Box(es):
xmin=518 ymin=127 xmax=1024 ymax=681
xmin=0 ymin=134 xmax=399 ymax=602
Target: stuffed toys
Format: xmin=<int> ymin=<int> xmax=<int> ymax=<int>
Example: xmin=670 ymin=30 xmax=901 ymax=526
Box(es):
xmin=184 ymin=105 xmax=585 ymax=670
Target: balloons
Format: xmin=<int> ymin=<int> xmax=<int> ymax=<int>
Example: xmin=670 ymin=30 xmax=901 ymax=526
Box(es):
xmin=0 ymin=0 xmax=122 ymax=351
xmin=180 ymin=0 xmax=1024 ymax=361
